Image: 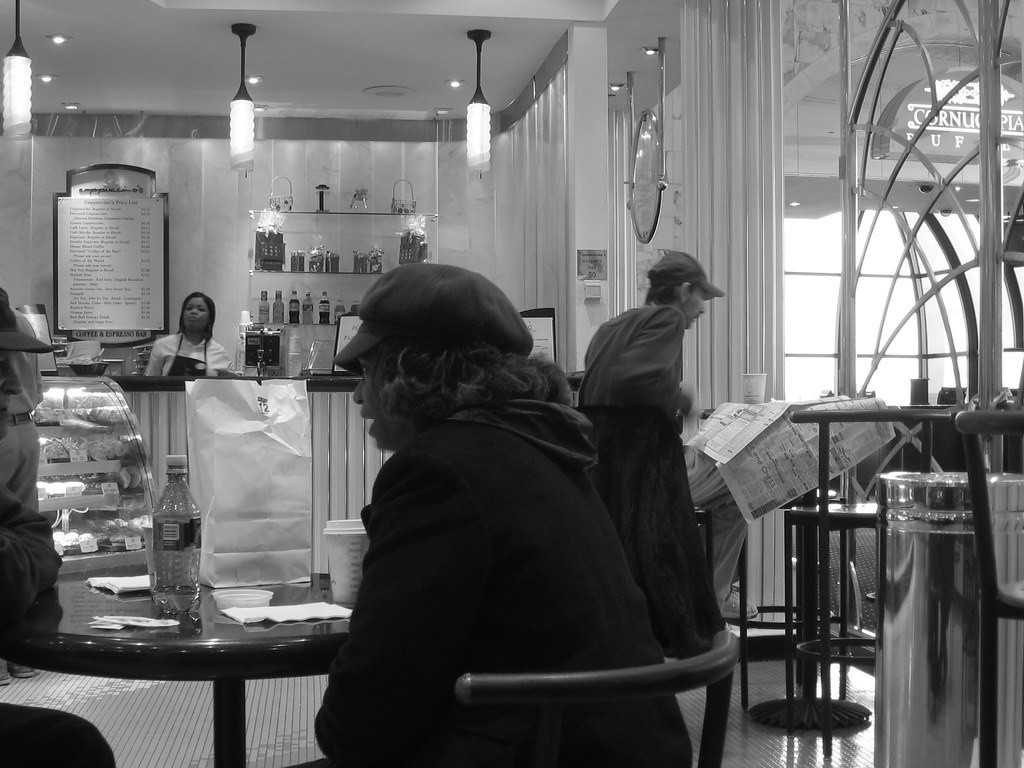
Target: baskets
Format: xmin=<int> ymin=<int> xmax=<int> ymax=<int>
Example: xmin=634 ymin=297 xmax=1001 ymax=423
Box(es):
xmin=268 ymin=175 xmax=293 ymax=210
xmin=390 ymin=179 xmax=417 ymax=214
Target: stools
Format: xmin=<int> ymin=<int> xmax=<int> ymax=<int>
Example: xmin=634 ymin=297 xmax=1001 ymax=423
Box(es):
xmin=790 ymin=410 xmax=952 ymax=758
xmin=694 ymin=493 xmax=847 ymax=711
xmin=951 ymin=409 xmax=1024 ymax=768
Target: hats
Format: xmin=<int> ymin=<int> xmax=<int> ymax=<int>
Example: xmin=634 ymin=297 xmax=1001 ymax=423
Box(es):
xmin=0 ymin=287 xmax=55 ymax=354
xmin=333 ymin=262 xmax=533 ymax=377
xmin=650 ymin=251 xmax=725 ymax=300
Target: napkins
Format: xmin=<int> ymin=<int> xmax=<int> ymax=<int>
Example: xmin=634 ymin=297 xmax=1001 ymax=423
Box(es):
xmin=85 ymin=575 xmax=150 ymax=594
xmin=218 ymin=601 xmax=353 ymax=624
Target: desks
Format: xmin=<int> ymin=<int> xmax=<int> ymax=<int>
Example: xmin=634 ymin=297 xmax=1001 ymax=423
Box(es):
xmin=699 ymin=404 xmax=969 ymax=733
xmin=0 ymin=563 xmax=357 ymax=768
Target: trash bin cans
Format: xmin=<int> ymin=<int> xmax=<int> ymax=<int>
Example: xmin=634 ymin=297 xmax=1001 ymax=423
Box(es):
xmin=874 ymin=470 xmax=1024 ymax=768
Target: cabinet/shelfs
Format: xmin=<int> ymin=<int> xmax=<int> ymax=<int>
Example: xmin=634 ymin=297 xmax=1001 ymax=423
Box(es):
xmin=27 ymin=374 xmax=158 ymax=574
xmin=248 ymin=209 xmax=438 ymax=326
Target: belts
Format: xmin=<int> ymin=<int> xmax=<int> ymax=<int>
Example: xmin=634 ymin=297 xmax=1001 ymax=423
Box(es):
xmin=9 ymin=413 xmax=30 ymax=421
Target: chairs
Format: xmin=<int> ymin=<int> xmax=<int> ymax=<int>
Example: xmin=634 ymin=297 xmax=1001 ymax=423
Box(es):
xmin=456 ymin=629 xmax=741 ymax=768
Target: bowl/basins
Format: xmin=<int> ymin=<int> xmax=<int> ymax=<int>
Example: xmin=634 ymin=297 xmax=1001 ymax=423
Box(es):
xmin=68 ymin=364 xmax=110 ymax=377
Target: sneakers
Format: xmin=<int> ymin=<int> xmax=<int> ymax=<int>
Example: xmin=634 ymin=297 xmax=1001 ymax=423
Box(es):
xmin=720 ymin=588 xmax=759 ymax=620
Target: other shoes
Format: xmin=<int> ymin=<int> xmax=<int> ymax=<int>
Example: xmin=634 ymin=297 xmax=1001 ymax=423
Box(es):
xmin=0 ymin=658 xmax=12 ymax=686
xmin=8 ymin=661 xmax=35 ymax=677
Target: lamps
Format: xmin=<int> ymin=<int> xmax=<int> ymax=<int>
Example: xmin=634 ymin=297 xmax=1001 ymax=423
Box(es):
xmin=34 ymin=74 xmax=58 ymax=82
xmin=609 ymin=83 xmax=626 ymax=91
xmin=245 ymin=76 xmax=263 ymax=84
xmin=230 ymin=23 xmax=256 ymax=171
xmin=642 ymin=47 xmax=659 ymax=55
xmin=466 ymin=29 xmax=491 ymax=173
xmin=447 ymin=79 xmax=465 ymax=88
xmin=3 ymin=0 xmax=32 ymax=141
xmin=46 ymin=34 xmax=74 ymax=44
xmin=60 ymin=102 xmax=81 ymax=110
xmin=254 ymin=104 xmax=268 ymax=111
xmin=434 ymin=108 xmax=453 ymax=115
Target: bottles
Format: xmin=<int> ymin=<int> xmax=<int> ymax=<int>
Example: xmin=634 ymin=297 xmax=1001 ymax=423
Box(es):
xmin=319 ymin=292 xmax=330 ymax=324
xmin=351 ymin=301 xmax=359 ymax=316
xmin=259 ymin=291 xmax=269 ymax=323
xmin=325 ymin=251 xmax=331 ymax=272
xmin=291 ymin=251 xmax=304 ymax=272
xmin=153 ymin=455 xmax=202 ymax=614
xmin=289 ymin=291 xmax=300 ymax=325
xmin=303 ymin=294 xmax=313 ymax=324
xmin=334 ymin=299 xmax=345 ymax=325
xmin=273 ymin=291 xmax=284 ymax=323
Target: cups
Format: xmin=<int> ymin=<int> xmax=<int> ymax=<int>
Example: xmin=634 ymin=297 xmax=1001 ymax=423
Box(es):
xmin=288 ymin=337 xmax=301 ymax=353
xmin=324 ymin=520 xmax=370 ymax=603
xmin=239 ymin=310 xmax=252 ymax=333
xmin=741 ymin=374 xmax=767 ymax=404
xmin=910 ymin=378 xmax=930 ymax=404
xmin=288 ymin=354 xmax=302 ymax=377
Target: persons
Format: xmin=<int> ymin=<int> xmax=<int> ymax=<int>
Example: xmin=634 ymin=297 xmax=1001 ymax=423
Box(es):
xmin=579 ymin=250 xmax=758 ymax=620
xmin=0 ymin=702 xmax=115 ymax=768
xmin=144 ymin=291 xmax=235 ymax=377
xmin=0 ymin=287 xmax=63 ymax=686
xmin=315 ymin=264 xmax=693 ymax=768
